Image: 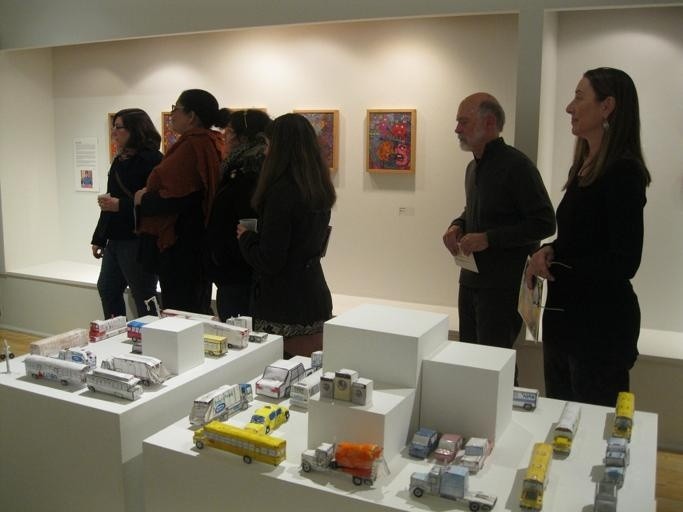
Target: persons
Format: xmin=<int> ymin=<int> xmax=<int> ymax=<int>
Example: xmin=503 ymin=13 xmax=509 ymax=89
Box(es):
xmin=524 ymin=67 xmax=652 ymax=407
xmin=132 ymin=89 xmax=232 ymax=318
xmin=439 ymin=90 xmax=554 ymax=386
xmin=235 ymin=113 xmax=336 ymax=360
xmin=90 ymin=107 xmax=168 ymax=320
xmin=204 ymin=110 xmax=275 ymax=325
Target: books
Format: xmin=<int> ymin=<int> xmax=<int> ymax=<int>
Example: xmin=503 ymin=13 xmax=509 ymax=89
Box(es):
xmin=513 ymin=253 xmax=543 ymax=344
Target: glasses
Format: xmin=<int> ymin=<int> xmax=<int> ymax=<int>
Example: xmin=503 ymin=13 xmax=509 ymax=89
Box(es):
xmin=536 ymin=262 xmax=572 ymax=311
xmin=114 ymin=124 xmax=125 ymax=130
xmin=172 ymin=104 xmax=184 ymax=112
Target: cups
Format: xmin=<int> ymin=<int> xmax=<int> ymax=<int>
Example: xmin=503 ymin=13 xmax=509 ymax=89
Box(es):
xmin=239 ymin=219 xmax=257 ymax=232
xmin=98 ymin=193 xmax=108 ymax=211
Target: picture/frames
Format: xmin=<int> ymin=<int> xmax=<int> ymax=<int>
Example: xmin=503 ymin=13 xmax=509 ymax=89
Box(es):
xmin=222 ymin=107 xmax=268 ymax=144
xmin=292 ymin=107 xmax=339 ymax=173
xmin=366 ymin=108 xmax=417 ymax=175
xmin=108 ymin=113 xmax=122 ymax=164
xmin=161 ymin=112 xmax=182 ymax=156
